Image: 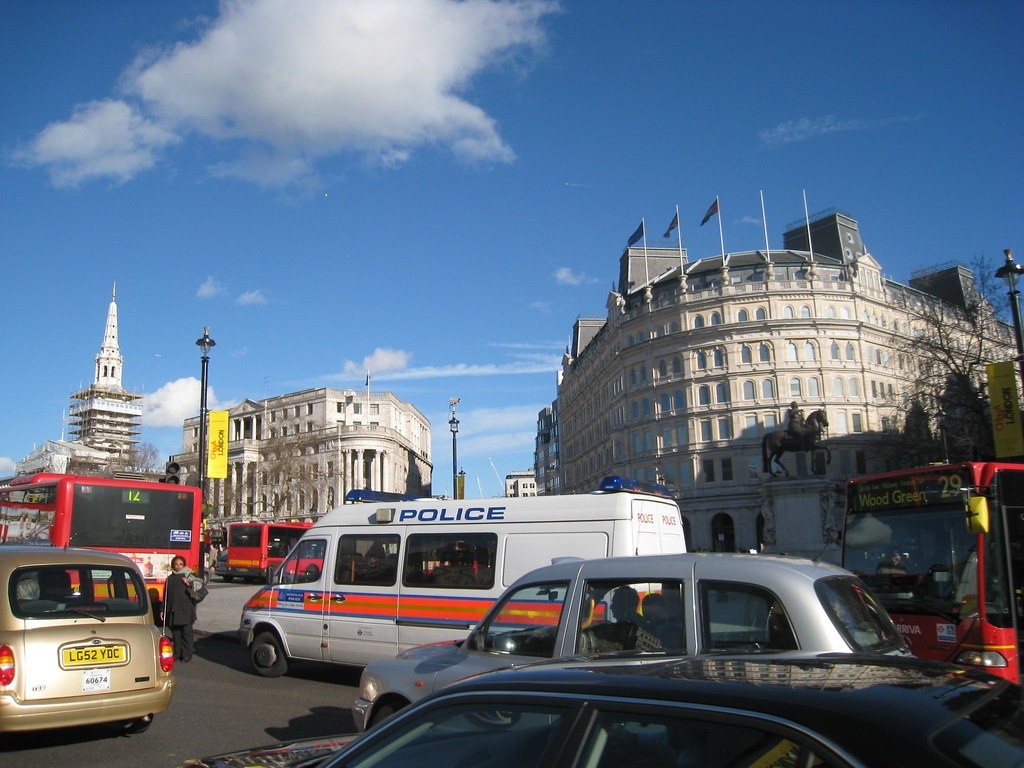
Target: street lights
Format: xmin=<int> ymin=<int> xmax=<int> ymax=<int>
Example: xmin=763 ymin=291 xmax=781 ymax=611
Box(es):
xmin=192 ymin=326 xmax=217 ymax=500
xmin=994 ymin=249 xmax=1024 ymax=379
xmin=448 ymin=416 xmax=460 ymax=501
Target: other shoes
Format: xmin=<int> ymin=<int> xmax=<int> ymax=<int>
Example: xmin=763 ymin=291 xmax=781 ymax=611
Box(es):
xmin=173 ymin=653 xmax=180 ymax=661
xmin=181 ymin=655 xmax=192 ymax=663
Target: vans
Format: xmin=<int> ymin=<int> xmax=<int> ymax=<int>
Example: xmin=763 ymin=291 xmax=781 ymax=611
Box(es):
xmin=235 ymin=471 xmax=691 ymax=678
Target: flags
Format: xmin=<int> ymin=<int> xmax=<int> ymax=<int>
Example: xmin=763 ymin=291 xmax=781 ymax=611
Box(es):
xmin=663 ymin=213 xmax=678 ymax=239
xmin=366 ymin=373 xmax=371 ymax=386
xmin=628 ymin=221 xmax=643 ymax=247
xmin=699 ymin=199 xmax=718 ymax=226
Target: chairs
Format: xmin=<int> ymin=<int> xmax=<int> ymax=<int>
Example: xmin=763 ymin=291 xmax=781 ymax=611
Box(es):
xmin=641 ymin=593 xmax=668 ymax=635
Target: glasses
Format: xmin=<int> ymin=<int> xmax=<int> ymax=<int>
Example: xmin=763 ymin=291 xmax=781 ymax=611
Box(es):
xmin=173 ymin=562 xmax=184 ymax=566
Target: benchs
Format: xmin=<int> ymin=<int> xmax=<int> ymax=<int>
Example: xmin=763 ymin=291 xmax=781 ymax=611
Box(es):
xmin=18 ymin=598 xmax=140 ymax=613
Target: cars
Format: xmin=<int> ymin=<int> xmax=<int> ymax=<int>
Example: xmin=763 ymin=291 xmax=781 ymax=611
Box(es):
xmin=351 ymin=549 xmax=919 ymax=746
xmin=214 ymin=547 xmax=227 ymax=581
xmin=177 ymin=653 xmax=1024 ymax=768
xmin=0 ymin=547 xmax=176 ymax=733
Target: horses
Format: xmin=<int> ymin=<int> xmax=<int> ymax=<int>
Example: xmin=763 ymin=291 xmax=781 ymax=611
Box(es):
xmin=761 ymin=407 xmax=832 ymax=477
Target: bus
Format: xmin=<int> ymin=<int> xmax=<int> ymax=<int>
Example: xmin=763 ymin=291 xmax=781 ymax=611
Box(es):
xmin=1 ymin=471 xmax=203 ymax=620
xmin=227 ymin=517 xmax=328 ymax=584
xmin=837 ymin=461 xmax=1024 ymax=688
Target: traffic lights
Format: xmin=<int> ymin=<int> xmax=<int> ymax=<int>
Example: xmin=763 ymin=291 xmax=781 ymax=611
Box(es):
xmin=163 ymin=461 xmax=181 ymax=486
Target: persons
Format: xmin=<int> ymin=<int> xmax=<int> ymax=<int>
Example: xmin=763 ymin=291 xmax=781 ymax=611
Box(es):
xmin=609 ymin=586 xmax=675 ymax=650
xmin=789 ymin=401 xmax=804 ymax=451
xmin=159 ymin=556 xmax=208 ymax=663
xmin=15 ymin=579 xmax=40 ymax=601
xmin=877 ymin=547 xmax=906 ymax=572
xmin=735 ymin=547 xmax=746 ymax=553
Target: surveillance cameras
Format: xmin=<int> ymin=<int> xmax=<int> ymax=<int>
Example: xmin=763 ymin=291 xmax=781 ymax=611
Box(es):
xmin=449 ymin=397 xmax=460 ymax=403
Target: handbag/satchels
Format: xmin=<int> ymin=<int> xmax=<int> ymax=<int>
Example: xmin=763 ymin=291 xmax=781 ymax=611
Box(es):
xmin=186 ymin=573 xmax=208 ymax=603
